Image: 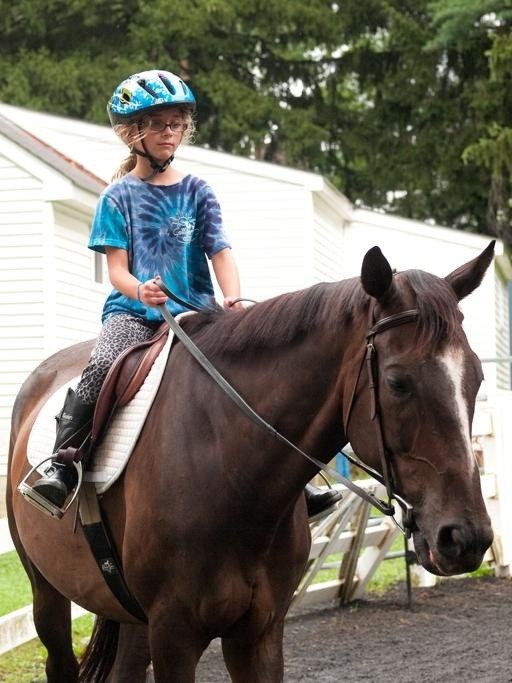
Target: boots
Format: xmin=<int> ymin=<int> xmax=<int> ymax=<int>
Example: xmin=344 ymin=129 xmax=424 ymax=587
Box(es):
xmin=307 ymin=482 xmax=342 ymax=517
xmin=33 ymin=387 xmax=96 ymax=507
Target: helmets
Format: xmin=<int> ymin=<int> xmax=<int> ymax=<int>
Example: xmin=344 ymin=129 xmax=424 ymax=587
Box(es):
xmin=107 ymin=70 xmax=196 ymax=124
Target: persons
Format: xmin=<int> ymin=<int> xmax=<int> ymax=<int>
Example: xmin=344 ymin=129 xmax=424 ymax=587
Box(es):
xmin=35 ymin=69 xmax=244 ymax=510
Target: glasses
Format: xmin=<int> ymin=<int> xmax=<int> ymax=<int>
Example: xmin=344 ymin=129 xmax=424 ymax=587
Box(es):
xmin=149 ymin=121 xmax=181 ymax=131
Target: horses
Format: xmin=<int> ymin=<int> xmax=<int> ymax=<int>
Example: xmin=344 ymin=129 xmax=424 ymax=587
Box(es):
xmin=4 ymin=239 xmax=496 ymax=683
